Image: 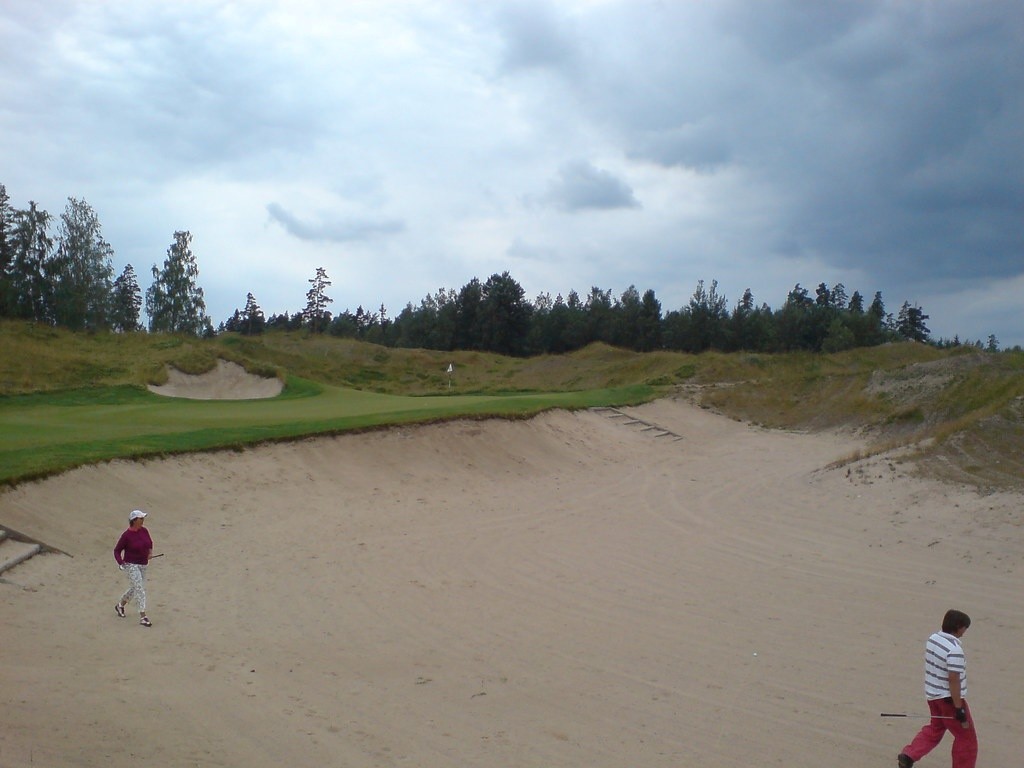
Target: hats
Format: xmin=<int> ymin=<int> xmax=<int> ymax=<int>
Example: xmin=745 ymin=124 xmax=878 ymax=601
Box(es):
xmin=128 ymin=510 xmax=147 ymax=521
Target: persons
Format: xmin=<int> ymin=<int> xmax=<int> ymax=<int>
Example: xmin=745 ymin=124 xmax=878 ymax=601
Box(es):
xmin=898 ymin=609 xmax=978 ymax=768
xmin=114 ymin=509 xmax=154 ymax=626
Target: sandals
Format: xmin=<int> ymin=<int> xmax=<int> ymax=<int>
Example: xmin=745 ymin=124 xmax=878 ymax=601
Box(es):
xmin=139 ymin=616 xmax=152 ymax=627
xmin=114 ymin=602 xmax=125 ymax=617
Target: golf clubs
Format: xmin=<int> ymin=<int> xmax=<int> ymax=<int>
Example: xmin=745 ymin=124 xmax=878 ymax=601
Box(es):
xmin=880 ymin=713 xmax=970 ymax=729
xmin=119 ymin=553 xmax=164 ymax=570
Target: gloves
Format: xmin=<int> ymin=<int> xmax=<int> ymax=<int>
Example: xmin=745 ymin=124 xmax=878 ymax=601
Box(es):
xmin=955 ymin=705 xmax=967 ymax=723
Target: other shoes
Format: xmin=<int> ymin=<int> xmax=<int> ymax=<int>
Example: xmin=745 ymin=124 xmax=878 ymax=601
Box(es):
xmin=898 ymin=753 xmax=914 ymax=768
